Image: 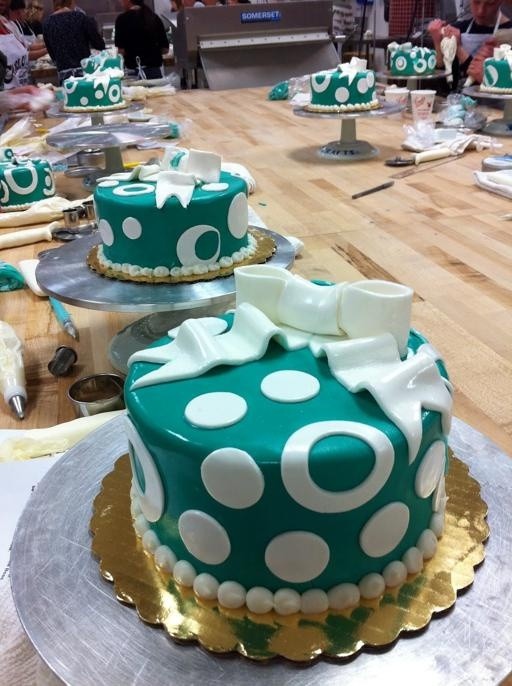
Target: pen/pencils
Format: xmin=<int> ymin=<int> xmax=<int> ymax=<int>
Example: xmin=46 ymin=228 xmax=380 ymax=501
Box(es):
xmin=352 ymin=181 xmax=395 ymax=199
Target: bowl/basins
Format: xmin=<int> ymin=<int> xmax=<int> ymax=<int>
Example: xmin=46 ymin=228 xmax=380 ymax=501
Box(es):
xmin=68 ymin=373 xmax=125 ymax=419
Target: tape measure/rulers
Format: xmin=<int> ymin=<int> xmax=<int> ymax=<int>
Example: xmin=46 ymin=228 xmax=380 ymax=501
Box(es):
xmin=388 ymin=154 xmax=466 ymax=178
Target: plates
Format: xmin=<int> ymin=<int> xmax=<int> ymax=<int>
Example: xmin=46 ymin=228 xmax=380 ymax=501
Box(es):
xmin=125 ymin=114 xmax=152 ymax=124
xmin=63 ymin=165 xmax=103 ymax=179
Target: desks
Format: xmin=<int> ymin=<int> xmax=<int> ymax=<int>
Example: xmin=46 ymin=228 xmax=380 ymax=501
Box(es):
xmin=2 ymin=81 xmax=512 ymax=686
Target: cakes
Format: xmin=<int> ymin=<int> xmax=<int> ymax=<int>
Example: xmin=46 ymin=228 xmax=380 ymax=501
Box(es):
xmin=387 ymin=42 xmax=437 ymax=76
xmin=62 ymin=72 xmax=126 ymax=110
xmin=0 ymin=156 xmax=56 ymax=209
xmin=123 ymin=264 xmax=454 ymax=616
xmin=308 ymin=56 xmax=378 ymax=110
xmin=480 ymin=44 xmax=512 ymax=92
xmin=80 ymin=47 xmax=124 ymax=79
xmin=92 ymin=145 xmax=259 ymax=278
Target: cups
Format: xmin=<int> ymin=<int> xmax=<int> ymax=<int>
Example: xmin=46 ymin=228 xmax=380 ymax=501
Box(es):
xmin=410 ymin=89 xmax=437 ymax=131
xmin=63 ymin=209 xmax=80 ymax=234
xmin=384 ymin=89 xmax=410 ymax=122
xmin=82 ymin=201 xmax=97 ymax=219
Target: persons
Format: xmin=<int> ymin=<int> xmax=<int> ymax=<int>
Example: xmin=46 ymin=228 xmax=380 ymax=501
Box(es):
xmin=426 ymin=0 xmax=512 ymax=99
xmin=40 ymin=0 xmax=107 ymax=88
xmin=114 ymin=0 xmax=169 ymax=87
xmin=466 ymin=35 xmax=502 ymax=85
xmin=0 ymin=0 xmax=45 ymax=89
xmin=193 ymin=0 xmax=251 ymax=6
xmin=161 ymin=0 xmax=184 ymax=34
xmin=0 ymin=83 xmax=56 ymax=135
